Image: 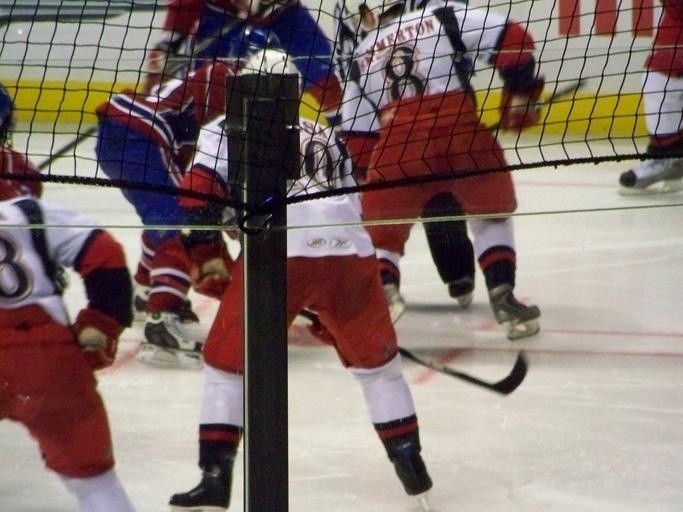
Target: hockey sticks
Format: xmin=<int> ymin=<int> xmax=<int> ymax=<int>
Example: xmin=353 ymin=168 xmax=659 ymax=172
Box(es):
xmin=202 ymin=258 xmax=529 ymax=395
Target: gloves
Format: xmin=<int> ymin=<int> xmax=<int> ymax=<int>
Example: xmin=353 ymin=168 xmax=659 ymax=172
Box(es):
xmin=496 ymin=62 xmax=544 ymax=134
xmin=182 ymin=236 xmax=239 ymax=302
xmin=67 ymin=305 xmax=128 ymax=372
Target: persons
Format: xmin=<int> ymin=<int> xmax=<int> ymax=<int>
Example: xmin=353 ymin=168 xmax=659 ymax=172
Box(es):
xmin=1 ymin=82 xmax=138 ymax=511
xmin=613 ymin=0 xmax=682 ymax=189
xmin=169 ymin=51 xmax=433 ymax=512
xmin=94 ymin=0 xmax=543 ymax=372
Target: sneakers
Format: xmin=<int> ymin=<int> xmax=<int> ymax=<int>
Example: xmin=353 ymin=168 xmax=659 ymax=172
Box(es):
xmin=392 ymin=443 xmax=435 ymax=498
xmin=620 ymin=153 xmax=683 ymax=192
xmin=144 ymin=297 xmax=207 ymax=353
xmin=447 ymin=270 xmax=477 ymax=300
xmin=166 ymin=458 xmax=235 ymax=509
xmin=490 ymin=283 xmax=542 ymax=328
